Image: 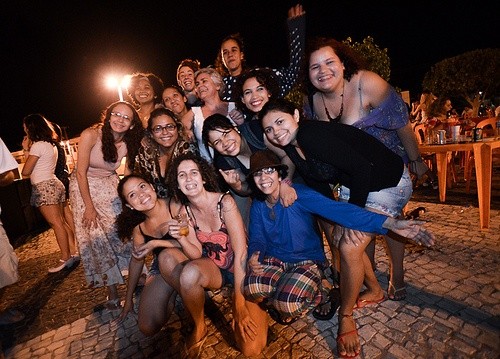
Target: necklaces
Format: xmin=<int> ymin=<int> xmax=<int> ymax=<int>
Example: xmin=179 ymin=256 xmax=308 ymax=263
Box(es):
xmin=320 ymin=80 xmax=345 ymax=123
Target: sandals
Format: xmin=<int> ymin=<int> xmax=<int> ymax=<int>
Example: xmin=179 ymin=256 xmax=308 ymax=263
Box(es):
xmin=312 ymin=288 xmax=340 ymax=321
xmin=279 ymin=313 xmax=300 ymax=325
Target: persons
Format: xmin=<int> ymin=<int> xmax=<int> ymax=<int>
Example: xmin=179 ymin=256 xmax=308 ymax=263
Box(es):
xmin=301 ymin=38 xmax=431 ymax=302
xmin=406 ymin=91 xmax=462 ymax=190
xmin=115 ymin=174 xmax=204 ymax=339
xmin=461 ymin=103 xmax=473 ymax=116
xmin=0 ymin=137 xmax=25 ymax=326
xmin=260 ymin=98 xmax=414 ymax=359
xmin=157 ymin=154 xmax=258 ymax=359
xmin=65 ymin=102 xmax=152 ymax=310
xmin=243 ymin=148 xmax=427 ymax=325
xmin=21 ymin=114 xmax=80 ymax=275
xmin=129 ymin=2 xmax=302 ymax=201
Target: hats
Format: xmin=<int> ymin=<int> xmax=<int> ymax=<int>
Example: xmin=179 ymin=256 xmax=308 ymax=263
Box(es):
xmin=245 ymin=152 xmax=289 ymax=182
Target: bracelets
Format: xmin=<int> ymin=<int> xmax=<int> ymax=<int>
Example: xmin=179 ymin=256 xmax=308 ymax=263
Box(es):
xmin=409 ymin=153 xmax=424 ymax=163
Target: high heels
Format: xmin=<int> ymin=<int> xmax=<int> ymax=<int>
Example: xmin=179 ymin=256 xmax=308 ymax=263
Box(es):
xmin=71 ymin=254 xmax=77 ymax=262
xmin=48 ymin=258 xmax=73 ymax=273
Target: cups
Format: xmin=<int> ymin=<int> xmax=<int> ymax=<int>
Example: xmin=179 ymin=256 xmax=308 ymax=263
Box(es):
xmin=428 ymin=130 xmax=434 ymax=144
xmin=438 ymin=130 xmax=447 ymax=144
xmin=174 ymin=214 xmax=189 ymax=236
xmin=452 ymin=125 xmax=460 ymax=142
xmin=472 ymin=128 xmax=480 ymax=141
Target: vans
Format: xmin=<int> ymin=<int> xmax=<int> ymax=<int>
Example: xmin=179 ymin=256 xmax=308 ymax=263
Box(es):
xmin=11 ymin=136 xmax=79 ymax=181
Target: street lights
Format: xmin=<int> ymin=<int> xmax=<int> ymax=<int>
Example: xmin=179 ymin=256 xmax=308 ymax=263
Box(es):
xmin=106 ymin=79 xmax=123 ymax=102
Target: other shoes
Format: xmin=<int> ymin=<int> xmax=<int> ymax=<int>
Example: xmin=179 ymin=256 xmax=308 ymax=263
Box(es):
xmin=109 ymin=298 xmax=121 ymax=306
xmin=133 ymin=303 xmax=139 ymax=314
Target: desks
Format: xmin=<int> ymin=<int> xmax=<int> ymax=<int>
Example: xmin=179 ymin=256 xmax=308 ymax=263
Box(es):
xmin=417 ymin=136 xmax=500 ymax=229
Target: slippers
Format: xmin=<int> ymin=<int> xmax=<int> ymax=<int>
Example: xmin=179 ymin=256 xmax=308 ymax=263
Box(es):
xmin=336 ymin=329 xmax=362 ymax=359
xmin=179 ymin=330 xmax=209 ymax=359
xmin=387 ymin=274 xmax=406 ymax=300
xmin=352 ymin=296 xmax=385 ymax=309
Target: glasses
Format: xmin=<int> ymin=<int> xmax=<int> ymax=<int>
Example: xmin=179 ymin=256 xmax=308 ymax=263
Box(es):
xmin=110 ymin=112 xmax=133 ymax=124
xmin=151 ymin=123 xmax=177 ymax=133
xmin=253 ymin=168 xmax=278 ymax=177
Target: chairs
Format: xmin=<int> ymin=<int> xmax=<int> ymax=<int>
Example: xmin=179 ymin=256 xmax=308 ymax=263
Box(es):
xmin=415 ymin=105 xmax=500 ymax=194
